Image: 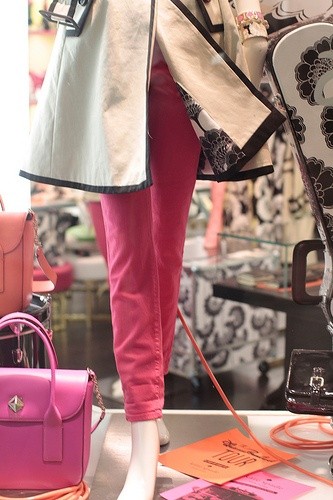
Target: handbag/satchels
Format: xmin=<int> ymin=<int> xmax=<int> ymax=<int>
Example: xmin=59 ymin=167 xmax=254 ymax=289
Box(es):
xmin=0 ymin=310 xmax=105 ymax=489
xmin=0 ymin=196 xmax=57 ymax=317
xmin=284 ymin=348 xmax=332 ymax=415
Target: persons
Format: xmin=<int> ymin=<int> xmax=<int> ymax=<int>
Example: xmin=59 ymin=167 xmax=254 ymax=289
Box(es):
xmin=16 ymin=0 xmax=290 ymax=500
